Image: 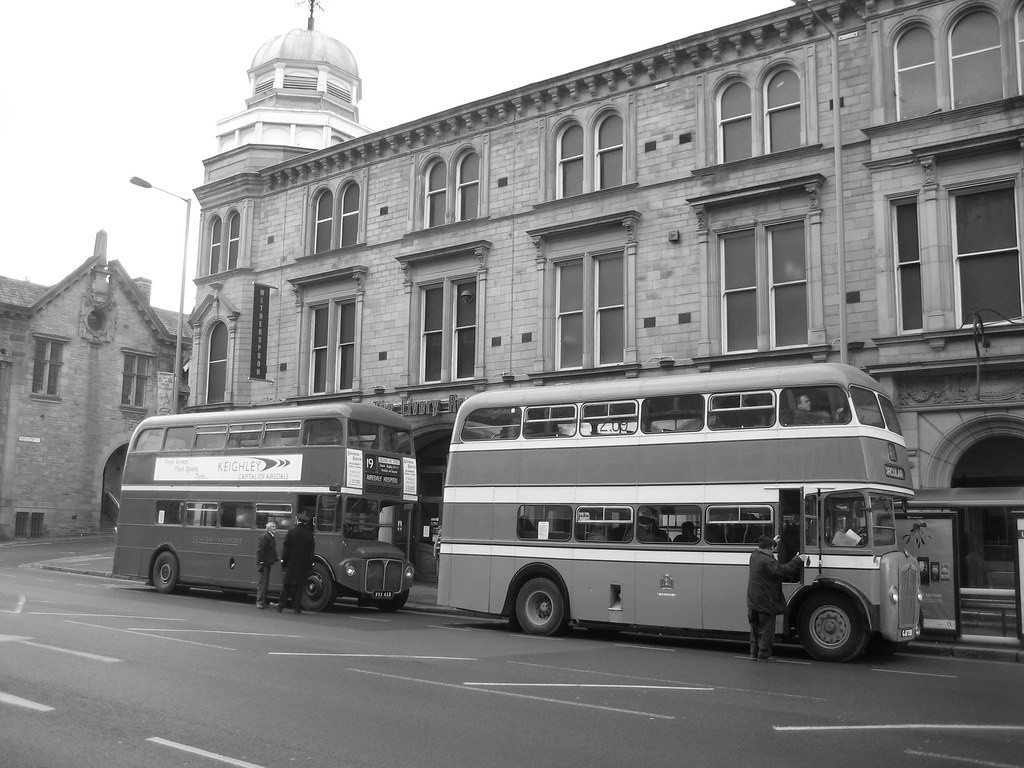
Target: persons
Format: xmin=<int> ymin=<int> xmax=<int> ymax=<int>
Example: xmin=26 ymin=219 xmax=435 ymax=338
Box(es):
xmin=270 ymin=514 xmax=315 ymax=615
xmin=673 ymin=521 xmax=700 ymax=541
xmin=746 ymin=534 xmax=807 ymax=663
xmin=256 ymin=522 xmax=278 ymax=609
xmin=795 ymin=394 xmax=830 ymax=424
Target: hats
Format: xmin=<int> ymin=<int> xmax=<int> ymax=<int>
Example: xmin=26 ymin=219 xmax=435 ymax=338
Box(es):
xmin=297 ymin=510 xmax=309 ymax=521
xmin=760 ymin=537 xmax=777 ymax=549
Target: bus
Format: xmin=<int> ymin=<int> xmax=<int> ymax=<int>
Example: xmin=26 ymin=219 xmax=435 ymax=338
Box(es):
xmin=113 ymin=402 xmax=420 ymax=611
xmin=436 ymin=363 xmax=927 ymax=664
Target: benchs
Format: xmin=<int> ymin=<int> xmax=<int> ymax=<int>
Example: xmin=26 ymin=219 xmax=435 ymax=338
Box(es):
xmin=958 ymin=587 xmax=1020 ymax=635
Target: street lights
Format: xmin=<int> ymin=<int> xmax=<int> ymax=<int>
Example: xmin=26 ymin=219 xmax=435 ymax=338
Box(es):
xmin=129 ymin=175 xmax=193 ymax=414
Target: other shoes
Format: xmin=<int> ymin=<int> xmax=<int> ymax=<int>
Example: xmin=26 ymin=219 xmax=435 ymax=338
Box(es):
xmin=757 ymin=658 xmax=776 ymax=663
xmin=751 ymin=655 xmax=757 ymax=660
xmin=270 ymin=602 xmax=282 ymax=613
xmin=294 ymin=609 xmax=301 ymax=614
xmin=256 ymin=603 xmax=264 ymax=608
